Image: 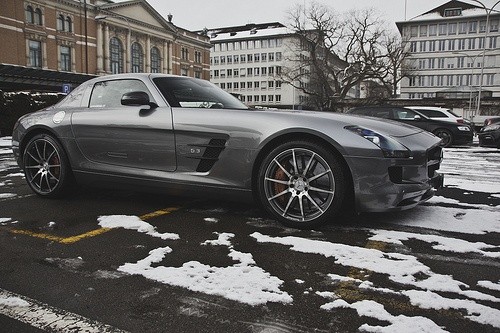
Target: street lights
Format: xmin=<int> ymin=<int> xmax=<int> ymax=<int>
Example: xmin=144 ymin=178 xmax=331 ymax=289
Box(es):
xmin=452 ymin=48 xmax=495 ymax=120
xmin=94 ymin=15 xmax=131 ymax=72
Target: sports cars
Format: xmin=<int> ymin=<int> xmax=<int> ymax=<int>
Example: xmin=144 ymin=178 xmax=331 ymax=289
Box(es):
xmin=12 ymin=73 xmax=443 ymax=229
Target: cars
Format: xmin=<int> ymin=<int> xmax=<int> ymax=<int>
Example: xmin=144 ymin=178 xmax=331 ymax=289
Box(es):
xmin=403 ymin=106 xmax=471 ymax=125
xmin=478 ymin=117 xmax=500 ymax=149
xmin=345 ymin=105 xmax=473 ymax=148
